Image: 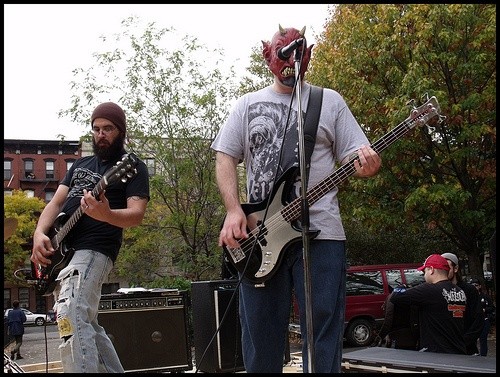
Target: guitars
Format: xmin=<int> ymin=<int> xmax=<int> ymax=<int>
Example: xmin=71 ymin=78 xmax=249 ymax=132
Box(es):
xmin=31 ymin=152 xmax=138 ymax=296
xmin=220 ymin=93 xmax=447 ymax=285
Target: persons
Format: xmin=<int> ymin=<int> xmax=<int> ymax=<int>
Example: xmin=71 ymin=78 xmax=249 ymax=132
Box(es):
xmin=389 ymin=253 xmax=483 ymax=356
xmin=211 ymin=29 xmax=382 ymax=373
xmin=7 ymin=300 xmax=27 ymax=360
xmin=30 ymin=102 xmax=151 ymax=373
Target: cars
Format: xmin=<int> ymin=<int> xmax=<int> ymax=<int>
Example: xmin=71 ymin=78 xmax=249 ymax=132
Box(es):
xmin=4 ymin=309 xmax=51 ymax=326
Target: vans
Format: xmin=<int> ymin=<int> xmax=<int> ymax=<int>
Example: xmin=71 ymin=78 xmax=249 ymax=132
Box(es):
xmin=294 ymin=263 xmax=426 ymax=346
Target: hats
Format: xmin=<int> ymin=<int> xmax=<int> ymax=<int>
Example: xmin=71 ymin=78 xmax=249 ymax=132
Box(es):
xmin=417 ymin=254 xmax=449 ymax=271
xmin=441 ymin=252 xmax=458 ymax=265
xmin=91 ymin=102 xmax=126 ymax=133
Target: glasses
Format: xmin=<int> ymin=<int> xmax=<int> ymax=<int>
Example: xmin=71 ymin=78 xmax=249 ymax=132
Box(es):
xmin=91 ymin=126 xmax=117 ymax=135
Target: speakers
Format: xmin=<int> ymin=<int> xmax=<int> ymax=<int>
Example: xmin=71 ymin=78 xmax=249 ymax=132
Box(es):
xmin=192 ymin=280 xmax=290 ymax=372
xmin=96 ymin=290 xmax=193 ymax=374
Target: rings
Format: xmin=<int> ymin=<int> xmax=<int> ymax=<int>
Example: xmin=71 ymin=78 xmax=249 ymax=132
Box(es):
xmin=85 ymin=205 xmax=88 ymax=211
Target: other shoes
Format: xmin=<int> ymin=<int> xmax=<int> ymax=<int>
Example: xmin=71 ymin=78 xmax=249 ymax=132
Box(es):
xmin=16 ymin=356 xmax=23 ymax=360
xmin=11 ymin=351 xmax=14 ymax=360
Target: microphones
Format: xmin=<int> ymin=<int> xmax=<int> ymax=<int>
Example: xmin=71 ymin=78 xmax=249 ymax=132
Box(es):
xmin=25 ymin=277 xmax=44 ymax=285
xmin=277 ymin=37 xmax=303 ymax=61
